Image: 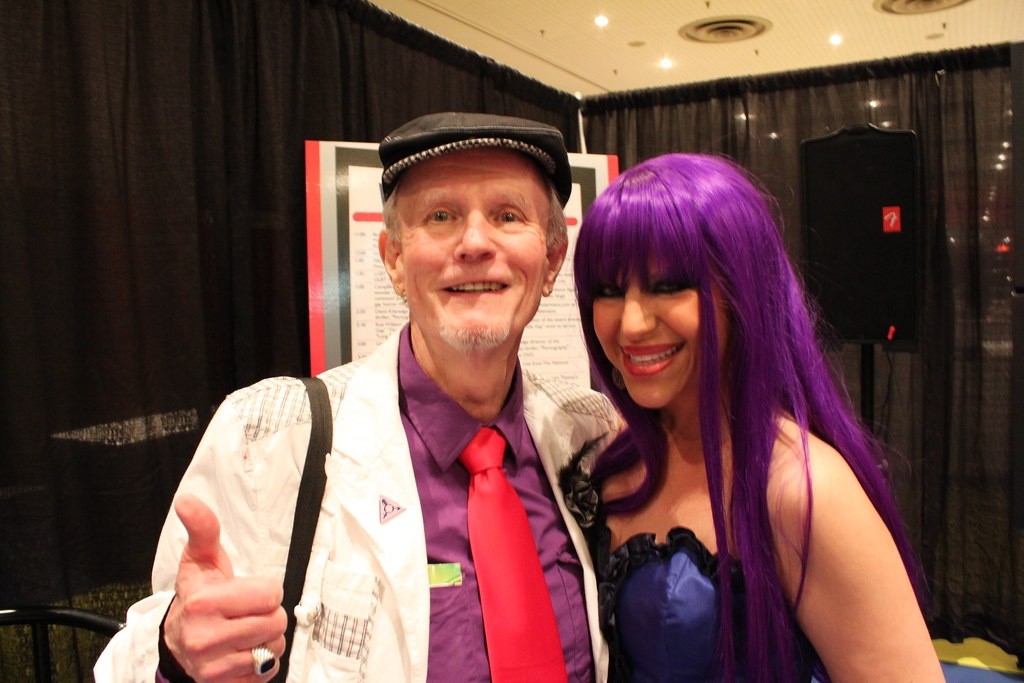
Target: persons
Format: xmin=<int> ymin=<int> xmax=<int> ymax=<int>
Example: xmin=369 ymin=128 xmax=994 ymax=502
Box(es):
xmin=92 ymin=109 xmax=629 ymax=683
xmin=558 ymin=153 xmax=948 ymax=682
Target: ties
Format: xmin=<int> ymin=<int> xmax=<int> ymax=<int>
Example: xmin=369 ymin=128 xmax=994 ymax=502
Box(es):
xmin=457 ymin=427 xmax=571 ymax=683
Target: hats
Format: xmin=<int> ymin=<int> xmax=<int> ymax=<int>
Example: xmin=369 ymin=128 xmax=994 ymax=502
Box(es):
xmin=378 ymin=110 xmax=574 ymax=208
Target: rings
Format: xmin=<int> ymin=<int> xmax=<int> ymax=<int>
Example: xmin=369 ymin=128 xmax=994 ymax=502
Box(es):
xmin=251 ymin=645 xmax=276 ymax=676
